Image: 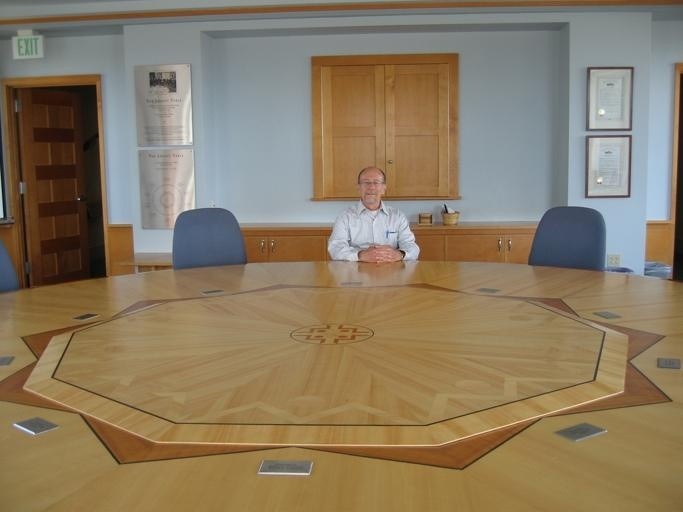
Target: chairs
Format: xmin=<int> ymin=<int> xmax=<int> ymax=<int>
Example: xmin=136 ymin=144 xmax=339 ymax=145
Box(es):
xmin=527 ymin=205 xmax=609 ymax=271
xmin=1 ymin=234 xmax=23 ymax=295
xmin=170 ymin=206 xmax=248 ymax=273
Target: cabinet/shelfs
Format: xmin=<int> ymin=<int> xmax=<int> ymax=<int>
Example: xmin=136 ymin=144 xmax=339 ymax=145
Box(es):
xmin=444 ymin=227 xmax=538 ymax=263
xmin=240 ymin=230 xmax=327 ymax=264
xmin=411 ymin=227 xmax=445 ymax=260
xmin=307 ymin=51 xmax=459 ymax=203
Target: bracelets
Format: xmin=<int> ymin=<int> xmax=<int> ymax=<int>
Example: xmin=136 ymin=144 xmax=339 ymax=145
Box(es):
xmin=399 ymin=252 xmax=403 ymax=261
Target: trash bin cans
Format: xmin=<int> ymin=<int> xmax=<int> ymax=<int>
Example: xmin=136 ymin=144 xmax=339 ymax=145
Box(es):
xmin=644 ymin=262 xmax=672 ymax=280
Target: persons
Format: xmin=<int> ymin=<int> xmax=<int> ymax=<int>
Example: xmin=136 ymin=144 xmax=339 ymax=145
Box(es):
xmin=326 ymin=167 xmax=420 ymax=266
xmin=149 ymin=78 xmax=175 ymax=88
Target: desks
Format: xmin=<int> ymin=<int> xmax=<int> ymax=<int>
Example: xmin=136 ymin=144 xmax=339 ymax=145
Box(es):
xmin=116 ymin=252 xmax=173 ymax=275
xmin=0 ymin=259 xmax=682 ymax=510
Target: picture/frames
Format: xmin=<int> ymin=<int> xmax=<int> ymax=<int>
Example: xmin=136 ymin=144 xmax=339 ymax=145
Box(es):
xmin=583 ymin=132 xmax=633 ymax=199
xmin=584 ymin=65 xmax=634 ymax=132
